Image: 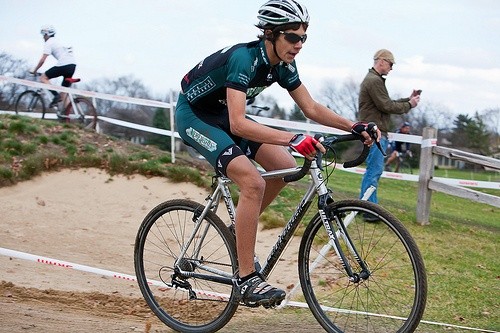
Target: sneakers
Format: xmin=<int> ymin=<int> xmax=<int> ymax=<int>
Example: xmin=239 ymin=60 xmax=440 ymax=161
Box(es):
xmin=230 ymin=275 xmax=286 ymax=309
xmin=228 ymin=224 xmax=261 ymax=273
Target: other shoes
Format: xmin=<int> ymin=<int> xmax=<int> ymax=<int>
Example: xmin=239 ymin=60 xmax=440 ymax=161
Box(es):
xmin=363 ymin=213 xmax=381 ymax=222
xmin=49 ymin=95 xmax=62 ymax=108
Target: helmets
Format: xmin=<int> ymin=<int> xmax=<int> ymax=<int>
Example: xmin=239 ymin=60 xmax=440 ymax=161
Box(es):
xmin=40 ymin=27 xmax=56 ymax=37
xmin=254 ymin=0 xmax=310 ymax=27
xmin=403 ymin=121 xmax=412 ymax=127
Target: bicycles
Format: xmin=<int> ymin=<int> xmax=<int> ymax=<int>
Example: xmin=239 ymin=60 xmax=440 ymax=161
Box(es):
xmin=133 ymin=122 xmax=428 ymax=333
xmin=14 ymin=70 xmax=96 ymax=132
xmin=384 ymin=151 xmax=413 ymax=174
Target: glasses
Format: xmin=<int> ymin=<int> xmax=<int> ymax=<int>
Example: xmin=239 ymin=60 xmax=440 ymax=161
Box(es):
xmin=279 ymin=31 xmax=307 ymax=43
xmin=384 ymin=59 xmax=393 ymax=67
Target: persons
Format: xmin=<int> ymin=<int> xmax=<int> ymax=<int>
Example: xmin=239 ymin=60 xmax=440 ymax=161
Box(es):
xmin=358 ymin=49 xmax=421 ymax=222
xmin=33 ymin=25 xmax=77 ymax=108
xmin=174 ymin=0 xmax=381 ymax=306
xmin=384 ymin=121 xmax=416 ymax=172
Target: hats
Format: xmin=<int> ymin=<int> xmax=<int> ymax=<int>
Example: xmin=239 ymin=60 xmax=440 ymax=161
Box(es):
xmin=374 ymin=49 xmax=396 ymax=64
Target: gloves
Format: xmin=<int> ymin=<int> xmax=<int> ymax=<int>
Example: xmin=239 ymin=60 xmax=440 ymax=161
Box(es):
xmin=405 ymin=150 xmax=413 ymax=158
